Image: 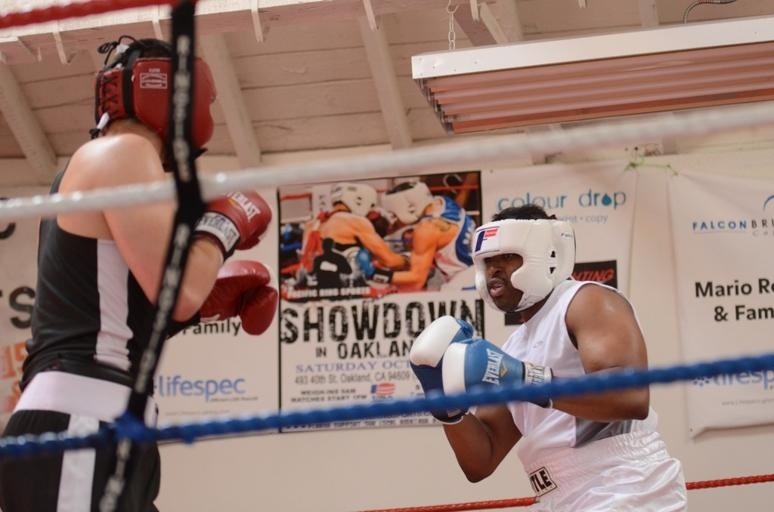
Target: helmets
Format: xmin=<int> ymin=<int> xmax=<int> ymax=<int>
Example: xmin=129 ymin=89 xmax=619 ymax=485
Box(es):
xmin=94 ymin=56 xmax=217 ymax=149
xmin=470 ymin=219 xmax=576 ymax=313
xmin=329 ymin=181 xmax=435 ymax=227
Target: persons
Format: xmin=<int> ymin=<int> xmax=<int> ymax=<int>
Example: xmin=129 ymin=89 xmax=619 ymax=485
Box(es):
xmin=295 ymin=182 xmax=435 ymax=297
xmin=1 ymin=30 xmax=278 ymax=510
xmin=407 ymin=203 xmax=688 ymax=511
xmin=354 ymin=180 xmax=477 ymax=289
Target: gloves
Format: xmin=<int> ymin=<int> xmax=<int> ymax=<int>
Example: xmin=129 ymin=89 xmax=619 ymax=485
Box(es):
xmin=408 ymin=314 xmax=553 ymax=426
xmin=192 ymin=190 xmax=272 ymax=260
xmin=347 ymin=247 xmax=377 ymax=278
xmin=198 ymin=259 xmax=279 ymax=335
